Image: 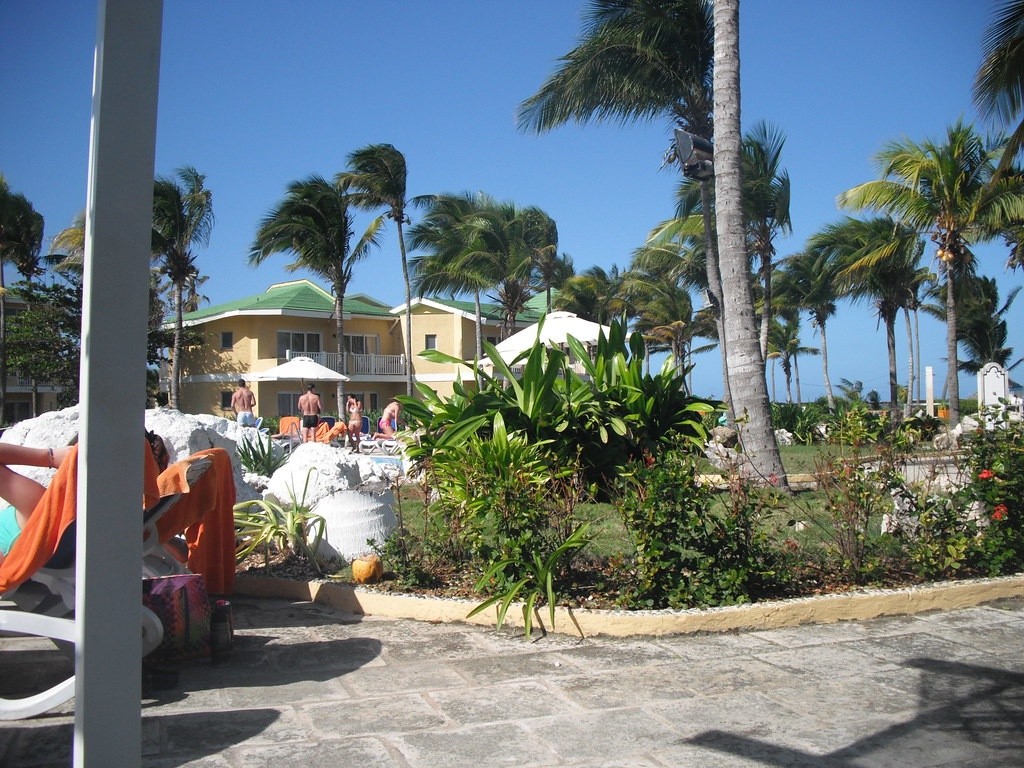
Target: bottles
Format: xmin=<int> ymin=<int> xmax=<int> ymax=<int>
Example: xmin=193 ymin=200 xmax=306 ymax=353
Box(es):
xmin=209 ymin=612 xmax=232 ymax=667
xmin=215 ymin=601 xmax=233 ymax=642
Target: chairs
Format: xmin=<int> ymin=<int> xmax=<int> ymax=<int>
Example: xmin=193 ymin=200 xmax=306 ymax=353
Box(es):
xmin=0 ymin=447 xmax=236 ymax=721
xmin=270 ymin=416 xmax=402 ymax=455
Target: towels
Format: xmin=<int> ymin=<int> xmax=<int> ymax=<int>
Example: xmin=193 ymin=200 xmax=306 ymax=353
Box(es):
xmin=0 ymin=439 xmax=159 ymax=596
xmin=278 ymin=417 xmax=347 ymax=444
xmin=159 ymin=447 xmax=236 ymax=596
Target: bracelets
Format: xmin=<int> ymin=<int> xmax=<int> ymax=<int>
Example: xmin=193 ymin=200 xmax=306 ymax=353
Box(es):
xmin=48 ymin=447 xmax=54 ymax=469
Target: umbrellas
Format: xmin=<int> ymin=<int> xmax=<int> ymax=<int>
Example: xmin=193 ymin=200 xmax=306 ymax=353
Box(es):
xmin=255 ymin=356 xmax=351 ymax=399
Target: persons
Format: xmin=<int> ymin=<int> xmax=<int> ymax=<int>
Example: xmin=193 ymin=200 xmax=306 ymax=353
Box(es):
xmin=347 ymin=394 xmax=364 ymax=451
xmin=0 ymin=430 xmax=75 ymax=568
xmin=231 ymin=379 xmax=256 ymax=427
xmin=298 ymin=383 xmax=321 ymax=444
xmin=372 ymin=399 xmax=401 ymax=439
